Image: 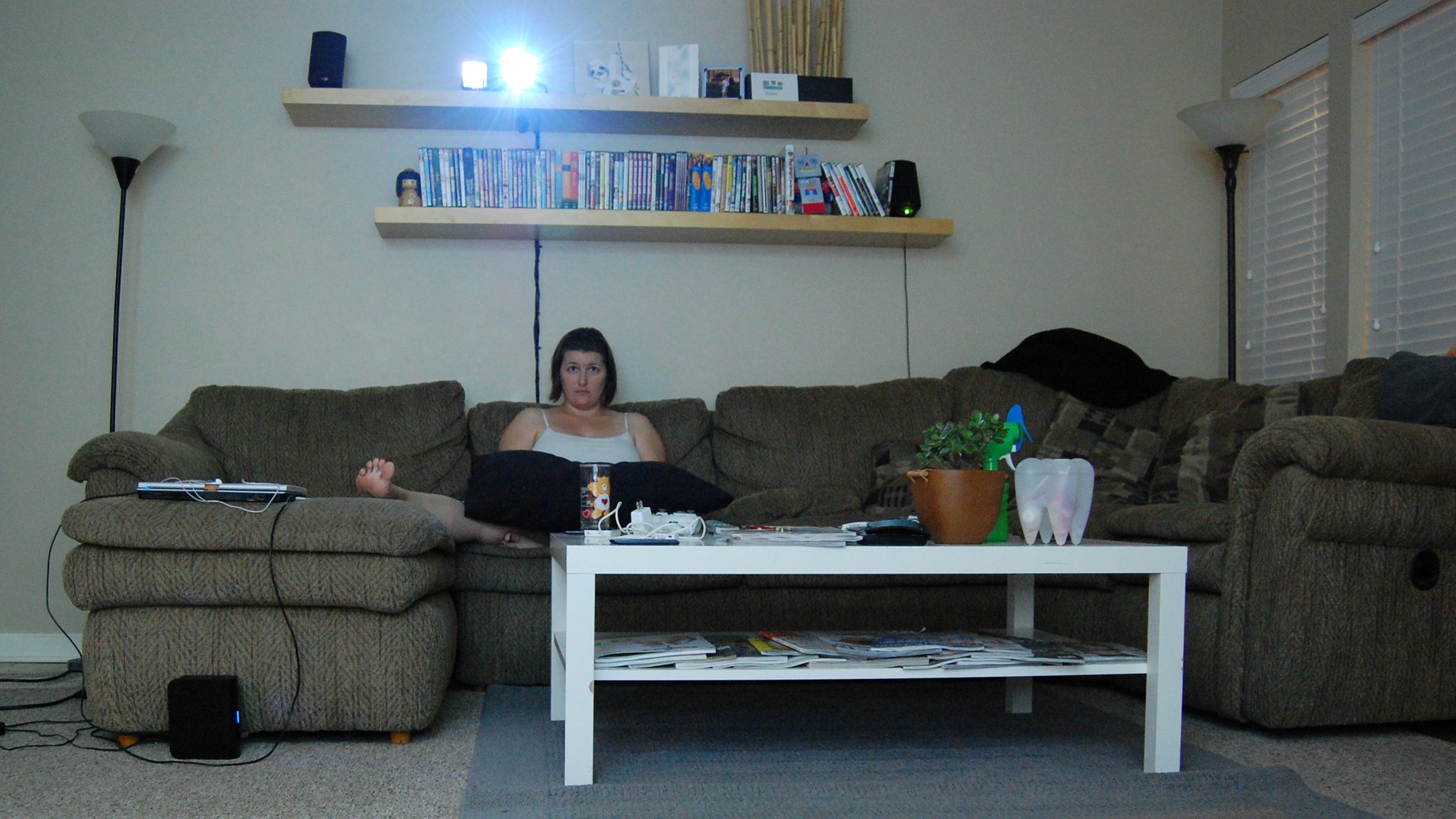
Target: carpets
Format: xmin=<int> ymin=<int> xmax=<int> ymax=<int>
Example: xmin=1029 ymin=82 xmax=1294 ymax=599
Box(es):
xmin=460 ymin=685 xmax=1377 ymax=819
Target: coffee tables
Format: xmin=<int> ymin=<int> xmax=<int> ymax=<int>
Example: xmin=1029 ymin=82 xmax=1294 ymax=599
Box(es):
xmin=549 ymin=532 xmax=1188 ymax=786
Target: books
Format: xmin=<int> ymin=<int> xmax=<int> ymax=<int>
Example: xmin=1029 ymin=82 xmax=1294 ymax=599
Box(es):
xmin=595 ymin=626 xmax=1147 ymax=670
xmin=724 ymin=520 xmax=866 ymax=548
xmin=417 ymin=146 xmax=885 ymax=218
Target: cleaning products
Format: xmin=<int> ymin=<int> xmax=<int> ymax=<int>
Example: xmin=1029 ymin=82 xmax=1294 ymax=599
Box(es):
xmin=981 ymin=404 xmax=1035 ymax=543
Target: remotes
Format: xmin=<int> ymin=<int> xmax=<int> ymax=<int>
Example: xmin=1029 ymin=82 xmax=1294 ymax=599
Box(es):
xmin=704 ymin=520 xmax=740 ymax=535
xmin=609 ymin=538 xmax=679 ymax=545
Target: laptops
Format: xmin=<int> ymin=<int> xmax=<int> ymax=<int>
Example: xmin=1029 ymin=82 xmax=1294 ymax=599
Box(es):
xmin=138 ymin=482 xmax=308 ymax=503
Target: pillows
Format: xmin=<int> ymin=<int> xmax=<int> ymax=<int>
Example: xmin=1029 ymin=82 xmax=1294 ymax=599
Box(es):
xmin=1033 ymin=395 xmax=1159 ymax=506
xmin=863 ymin=439 xmax=973 ymax=512
xmin=1145 ymin=385 xmax=1298 ymax=504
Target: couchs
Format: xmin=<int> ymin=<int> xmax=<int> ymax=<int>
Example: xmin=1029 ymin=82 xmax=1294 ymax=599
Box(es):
xmin=61 ymin=352 xmax=1456 ymax=745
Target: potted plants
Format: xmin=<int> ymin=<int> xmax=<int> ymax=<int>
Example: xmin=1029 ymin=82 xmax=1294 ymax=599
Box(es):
xmin=910 ymin=411 xmax=1009 ymax=544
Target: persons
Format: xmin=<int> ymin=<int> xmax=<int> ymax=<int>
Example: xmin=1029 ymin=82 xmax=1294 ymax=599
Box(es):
xmin=395 ymin=168 xmax=423 ymax=207
xmin=355 ymin=326 xmax=667 ymax=548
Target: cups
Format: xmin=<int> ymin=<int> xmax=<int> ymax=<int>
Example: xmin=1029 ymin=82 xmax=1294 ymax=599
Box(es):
xmin=579 ymin=464 xmax=611 ymax=529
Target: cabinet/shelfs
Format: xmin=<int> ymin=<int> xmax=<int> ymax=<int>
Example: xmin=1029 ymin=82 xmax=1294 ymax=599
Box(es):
xmin=282 ymin=87 xmax=954 ymax=248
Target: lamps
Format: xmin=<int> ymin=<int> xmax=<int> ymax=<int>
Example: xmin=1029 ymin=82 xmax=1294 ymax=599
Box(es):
xmin=1177 ymin=98 xmax=1283 ymax=381
xmin=78 ymin=110 xmax=177 ymax=432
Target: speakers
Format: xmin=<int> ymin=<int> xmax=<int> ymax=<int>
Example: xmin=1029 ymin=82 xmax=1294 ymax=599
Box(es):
xmin=307 ymin=30 xmax=346 ymax=89
xmin=876 ymin=160 xmax=922 ymax=217
